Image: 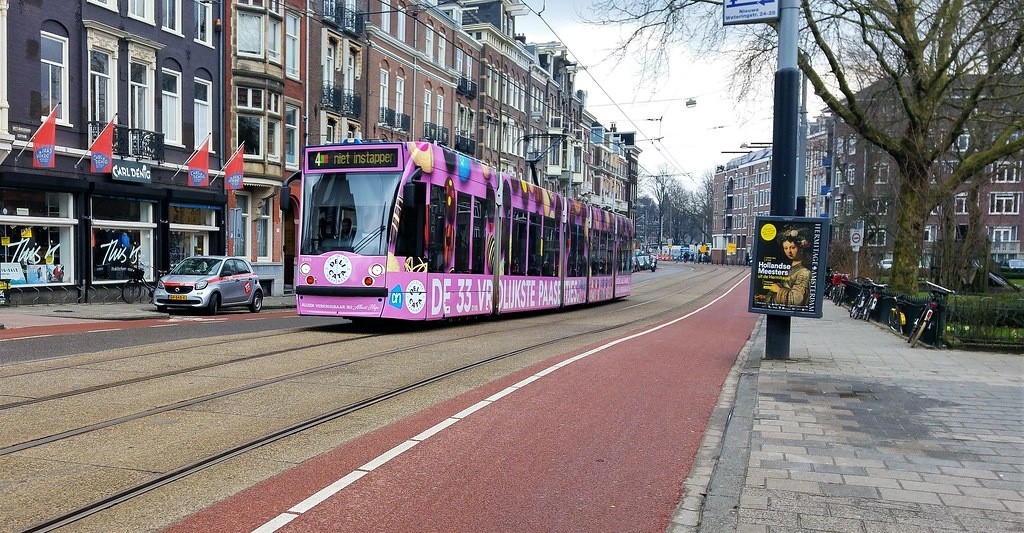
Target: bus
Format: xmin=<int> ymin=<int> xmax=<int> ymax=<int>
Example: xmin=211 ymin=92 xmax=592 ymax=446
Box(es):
xmin=279 ymin=133 xmax=635 ymax=322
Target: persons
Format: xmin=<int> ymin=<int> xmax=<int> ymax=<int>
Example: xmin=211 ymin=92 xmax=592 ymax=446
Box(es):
xmin=341 ymin=218 xmax=355 ymax=239
xmin=118 ymin=233 xmax=130 ymax=278
xmin=744 ymin=252 xmax=749 ymax=265
xmin=360 ymin=214 xmax=376 ymax=238
xmin=680 ymin=251 xmax=710 ymax=264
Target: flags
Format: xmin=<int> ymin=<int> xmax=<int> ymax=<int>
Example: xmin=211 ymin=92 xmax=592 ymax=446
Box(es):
xmin=33 ymin=110 xmax=55 ymax=168
xmin=91 ymin=119 xmax=113 ymax=172
xmin=225 ymin=146 xmax=244 ymax=189
xmin=188 ymin=141 xmax=209 ymax=186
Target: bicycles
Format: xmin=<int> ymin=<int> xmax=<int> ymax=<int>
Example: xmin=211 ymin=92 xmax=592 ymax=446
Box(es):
xmin=823 ymin=269 xmax=852 ymax=307
xmin=849 ymin=276 xmax=888 ymax=321
xmin=906 ymin=280 xmax=955 ymax=349
xmin=884 ymin=294 xmax=907 ymax=336
xmin=122 ymin=258 xmax=168 ymax=304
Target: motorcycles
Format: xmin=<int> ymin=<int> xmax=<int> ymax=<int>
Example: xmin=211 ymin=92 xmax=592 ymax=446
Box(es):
xmin=649 ymin=259 xmax=658 ymax=272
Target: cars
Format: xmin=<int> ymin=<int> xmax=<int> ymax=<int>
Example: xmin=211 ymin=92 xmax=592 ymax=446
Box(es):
xmin=999 ymin=259 xmax=1024 ymax=273
xmin=630 ymin=255 xmax=652 ymax=272
xmin=877 ymin=259 xmax=893 ymax=271
xmin=152 ymin=256 xmax=265 ymax=316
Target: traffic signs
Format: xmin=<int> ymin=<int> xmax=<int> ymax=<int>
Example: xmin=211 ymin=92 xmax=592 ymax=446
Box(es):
xmin=722 ymin=0 xmax=781 ymax=26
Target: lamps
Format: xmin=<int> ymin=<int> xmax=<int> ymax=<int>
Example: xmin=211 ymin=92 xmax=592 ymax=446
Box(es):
xmin=685 ymin=98 xmax=696 ymax=108
xmin=740 ymin=143 xmax=747 ymax=149
xmin=216 ymin=19 xmax=221 ymax=32
